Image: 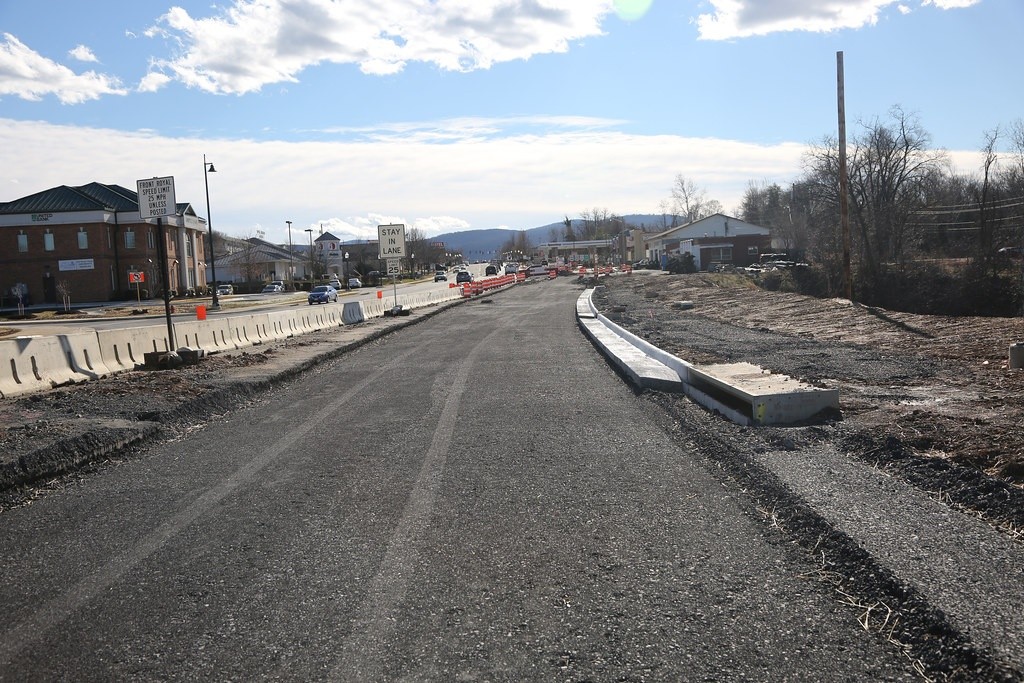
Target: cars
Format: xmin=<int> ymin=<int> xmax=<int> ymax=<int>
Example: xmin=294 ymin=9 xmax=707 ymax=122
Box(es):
xmin=996 ymin=247 xmax=1020 ymax=257
xmin=262 ymin=284 xmax=281 ymax=293
xmin=272 ymin=280 xmax=285 ymax=291
xmin=347 ymin=278 xmax=362 ymax=288
xmin=632 ymin=249 xmax=809 ymax=275
xmin=329 ymin=279 xmax=341 ymax=290
xmin=435 ymin=271 xmax=447 ymax=282
xmin=457 ymin=271 xmax=475 ymax=284
xmin=308 ymin=285 xmax=338 ymax=305
xmin=218 ymin=284 xmax=234 ymax=294
xmin=367 ymin=256 xmax=549 ymax=278
xmin=202 ymin=286 xmax=220 ymax=296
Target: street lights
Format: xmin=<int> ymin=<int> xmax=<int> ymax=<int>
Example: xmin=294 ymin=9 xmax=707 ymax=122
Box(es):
xmin=445 ymin=252 xmax=462 ymax=272
xmin=305 ymin=229 xmax=315 ymax=290
xmin=411 ymin=253 xmax=415 ymax=280
xmin=286 ymin=221 xmax=298 ymax=290
xmin=378 ymin=254 xmax=383 ymax=287
xmin=203 ymin=152 xmax=221 ymax=307
xmin=344 ymin=251 xmax=351 ymax=291
xmin=502 ymin=250 xmax=523 ymax=265
